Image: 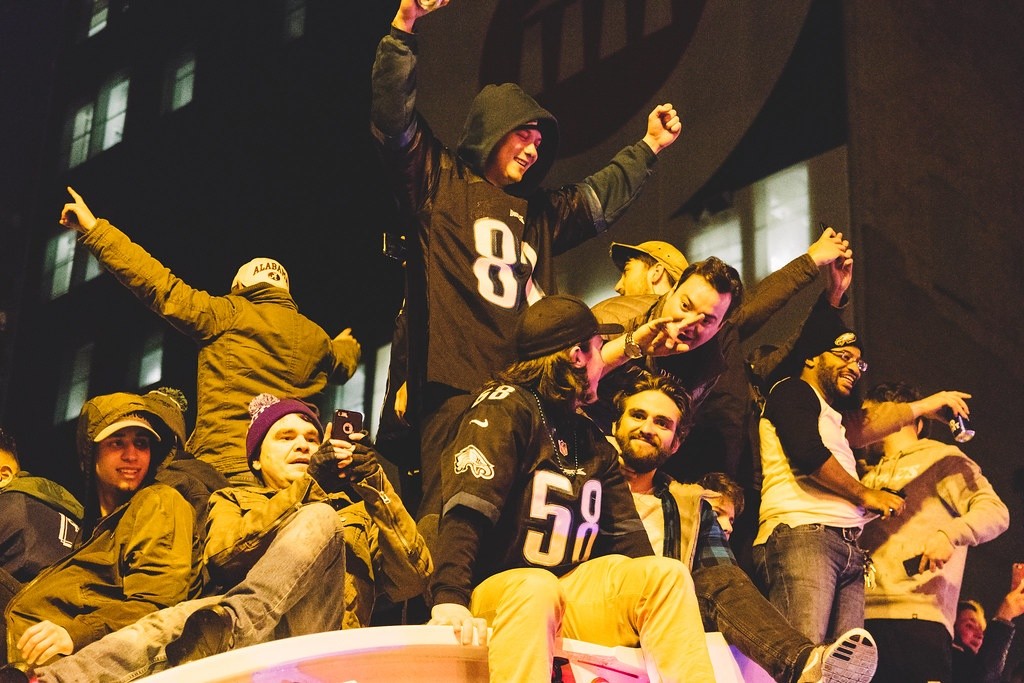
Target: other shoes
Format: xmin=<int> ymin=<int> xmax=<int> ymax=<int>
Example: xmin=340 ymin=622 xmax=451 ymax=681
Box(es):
xmin=164 ymin=604 xmax=236 ymax=667
xmin=0 ymin=662 xmax=37 ymax=683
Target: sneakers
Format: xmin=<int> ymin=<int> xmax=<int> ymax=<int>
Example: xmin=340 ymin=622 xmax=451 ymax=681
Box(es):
xmin=793 ymin=627 xmax=878 ymax=683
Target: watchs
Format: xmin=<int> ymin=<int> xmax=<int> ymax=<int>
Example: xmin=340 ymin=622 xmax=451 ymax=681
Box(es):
xmin=624 ymin=332 xmax=645 ymax=360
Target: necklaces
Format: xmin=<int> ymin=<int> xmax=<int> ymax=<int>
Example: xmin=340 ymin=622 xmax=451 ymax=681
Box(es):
xmin=530 ymin=389 xmax=580 ymax=476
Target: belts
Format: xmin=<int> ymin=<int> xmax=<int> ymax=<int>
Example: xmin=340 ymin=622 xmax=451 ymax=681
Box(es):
xmin=812 ymin=523 xmax=855 ymax=542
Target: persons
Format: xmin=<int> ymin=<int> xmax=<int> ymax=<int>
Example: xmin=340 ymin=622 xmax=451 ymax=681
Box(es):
xmin=368 ymin=0 xmax=680 ymax=582
xmin=0 ymin=183 xmax=1024 ymax=682
xmin=428 ymin=296 xmax=714 ymax=681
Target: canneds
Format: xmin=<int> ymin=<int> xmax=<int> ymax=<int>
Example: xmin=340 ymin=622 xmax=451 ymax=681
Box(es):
xmin=944 ymin=407 xmax=975 ymax=442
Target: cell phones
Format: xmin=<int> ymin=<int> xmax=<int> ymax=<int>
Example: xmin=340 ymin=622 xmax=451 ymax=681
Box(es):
xmin=902 ymin=554 xmax=929 ymax=578
xmin=330 ymin=409 xmax=362 ymax=445
xmin=1011 ymin=563 xmax=1024 ymax=593
xmin=819 ymin=222 xmax=843 ymax=267
xmin=868 ymin=487 xmax=906 ymax=516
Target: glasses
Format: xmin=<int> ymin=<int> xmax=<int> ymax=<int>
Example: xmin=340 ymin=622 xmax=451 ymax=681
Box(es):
xmin=826 ymin=350 xmax=868 ymax=371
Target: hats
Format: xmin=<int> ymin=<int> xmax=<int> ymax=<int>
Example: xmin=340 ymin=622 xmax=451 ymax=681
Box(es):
xmin=94 ymin=411 xmax=161 ymax=443
xmin=611 ymin=239 xmax=689 ymax=283
xmin=231 ymin=258 xmax=290 ymax=293
xmin=140 ymin=387 xmax=188 ymax=450
xmin=515 ymin=295 xmax=625 ymax=363
xmin=798 ymin=312 xmax=865 ymax=366
xmin=246 ymin=393 xmax=325 ymax=480
